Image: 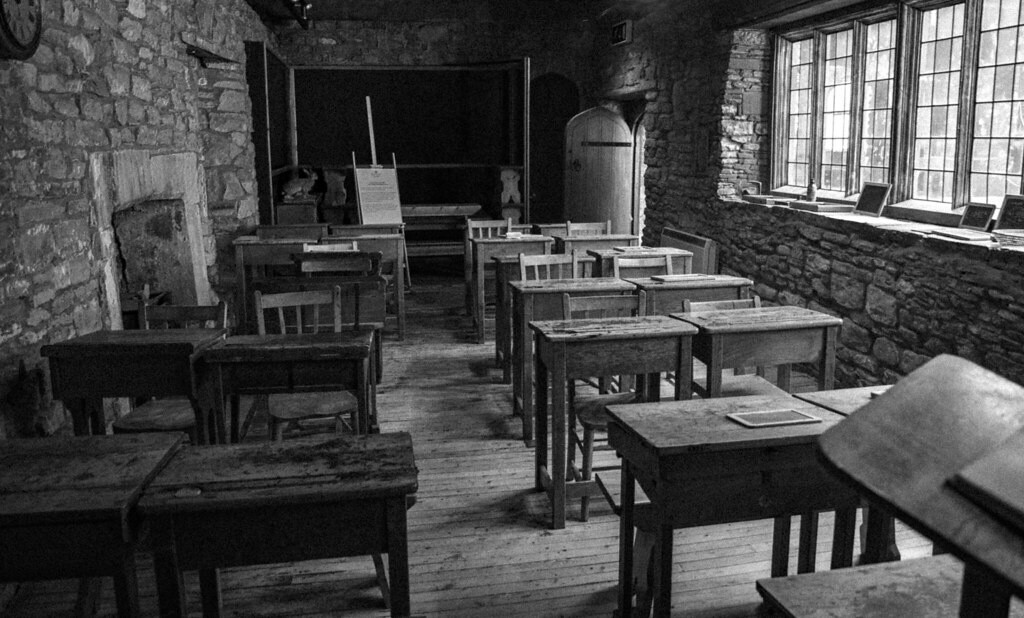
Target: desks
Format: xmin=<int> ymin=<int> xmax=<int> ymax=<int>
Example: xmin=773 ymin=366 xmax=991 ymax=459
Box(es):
xmin=490 ymin=254 xmax=596 ymax=384
xmin=138 ymin=432 xmax=418 ymax=618
xmin=0 ymin=432 xmax=190 ymax=618
xmin=468 ymin=236 xmax=554 ymax=343
xmin=669 ymin=305 xmax=843 ymax=398
xmin=329 ymin=222 xmax=406 ymax=236
xmin=551 ymin=234 xmax=639 ymax=255
xmin=255 ymin=223 xmax=329 ymax=245
xmin=233 ymin=236 xmax=320 ymax=335
xmin=792 ymin=384 xmax=896 ymax=575
xmin=321 ymin=234 xmax=406 ymax=341
xmin=605 ymin=396 xmax=890 ymax=618
xmin=40 ymin=329 xmax=227 ymax=446
xmin=402 ymin=205 xmax=481 ymax=282
xmin=586 ymin=247 xmax=694 ymax=278
xmin=535 ymin=223 xmax=567 ymax=279
xmin=205 ymin=331 xmax=376 ymax=445
xmin=756 ymin=553 xmax=1024 ymax=618
xmin=508 ymin=281 xmax=636 ymax=444
xmin=529 ymin=315 xmax=698 ymax=529
xmin=622 ymin=274 xmax=754 ymax=379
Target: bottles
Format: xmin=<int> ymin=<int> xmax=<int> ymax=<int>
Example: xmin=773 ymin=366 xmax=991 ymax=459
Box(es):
xmin=806 ymin=178 xmax=817 ymax=202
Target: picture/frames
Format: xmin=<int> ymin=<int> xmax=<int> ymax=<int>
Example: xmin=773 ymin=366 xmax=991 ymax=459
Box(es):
xmin=958 ymin=202 xmax=995 ymax=232
xmin=852 ymin=182 xmax=892 ymax=217
xmin=993 ymin=194 xmax=1024 ymax=230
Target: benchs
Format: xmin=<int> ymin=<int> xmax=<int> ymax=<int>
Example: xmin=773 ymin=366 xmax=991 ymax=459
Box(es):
xmin=595 ymin=474 xmax=656 ymax=618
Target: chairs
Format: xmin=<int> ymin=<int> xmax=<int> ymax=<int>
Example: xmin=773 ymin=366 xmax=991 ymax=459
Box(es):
xmin=561 ymin=291 xmax=646 ymax=521
xmin=518 ymin=249 xmax=577 ymax=280
xmin=566 ymin=220 xmax=611 ymax=236
xmin=613 ymin=254 xmax=673 ymax=278
xmin=681 ymin=296 xmax=792 ymax=398
xmin=467 ymin=217 xmax=512 ymax=315
xmin=112 ymin=302 xmax=227 ymax=445
xmin=253 ymin=286 xmax=360 ymax=441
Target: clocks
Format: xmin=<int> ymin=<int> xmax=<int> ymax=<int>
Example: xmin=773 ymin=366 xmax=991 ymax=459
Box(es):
xmin=0 ymin=0 xmax=43 ymax=60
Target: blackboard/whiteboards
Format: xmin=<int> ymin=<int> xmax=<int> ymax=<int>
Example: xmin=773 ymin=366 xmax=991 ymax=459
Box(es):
xmin=992 ymin=193 xmax=1024 ymax=229
xmin=958 ymin=200 xmax=996 ymax=232
xmin=287 ymin=64 xmax=515 ymax=170
xmin=852 ymin=180 xmax=893 ymax=218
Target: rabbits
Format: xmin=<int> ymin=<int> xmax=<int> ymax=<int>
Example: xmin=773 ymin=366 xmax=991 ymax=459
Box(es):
xmin=282 ymin=167 xmax=318 ymax=200
